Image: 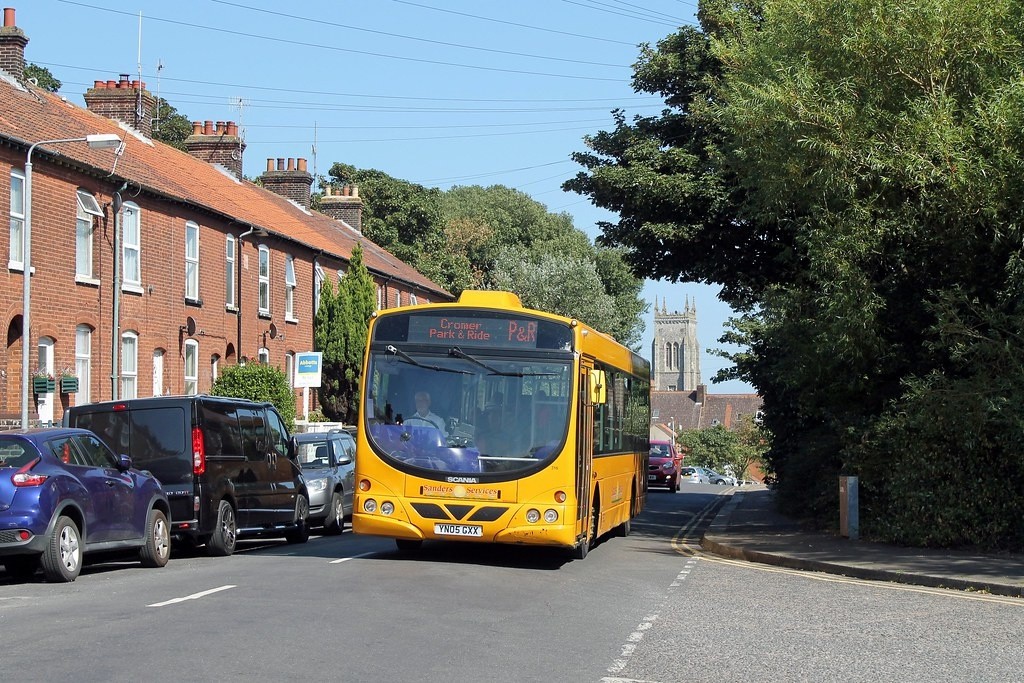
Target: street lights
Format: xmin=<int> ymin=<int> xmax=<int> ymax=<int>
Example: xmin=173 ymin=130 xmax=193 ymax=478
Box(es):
xmin=670 ymin=416 xmax=675 ymax=446
xmin=19 ymin=133 xmax=121 ymax=428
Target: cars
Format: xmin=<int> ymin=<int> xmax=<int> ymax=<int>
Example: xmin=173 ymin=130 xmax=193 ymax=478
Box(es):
xmin=645 ymin=440 xmax=684 ymax=494
xmin=1 ymin=429 xmax=174 ymax=582
xmin=703 ymin=467 xmax=734 ymax=487
xmin=681 ymin=467 xmax=710 ymax=485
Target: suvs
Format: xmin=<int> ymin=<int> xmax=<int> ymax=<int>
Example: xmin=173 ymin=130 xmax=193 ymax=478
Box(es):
xmin=290 ymin=428 xmax=357 ymax=536
xmin=59 ymin=394 xmax=310 ymax=558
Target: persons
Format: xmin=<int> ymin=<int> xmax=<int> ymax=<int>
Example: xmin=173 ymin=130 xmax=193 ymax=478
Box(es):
xmin=401 ymin=391 xmax=446 ymax=437
xmin=469 ymin=390 xmax=548 ymax=433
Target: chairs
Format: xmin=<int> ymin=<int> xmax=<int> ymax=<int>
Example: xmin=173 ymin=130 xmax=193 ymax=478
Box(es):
xmin=312 ymin=447 xmax=328 ymax=465
xmin=592 ymin=423 xmax=649 ymax=456
xmin=651 ymin=453 xmax=663 ymax=457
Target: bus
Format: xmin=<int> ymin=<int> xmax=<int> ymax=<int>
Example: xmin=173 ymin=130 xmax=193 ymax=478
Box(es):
xmin=351 ymin=290 xmax=651 ymax=561
